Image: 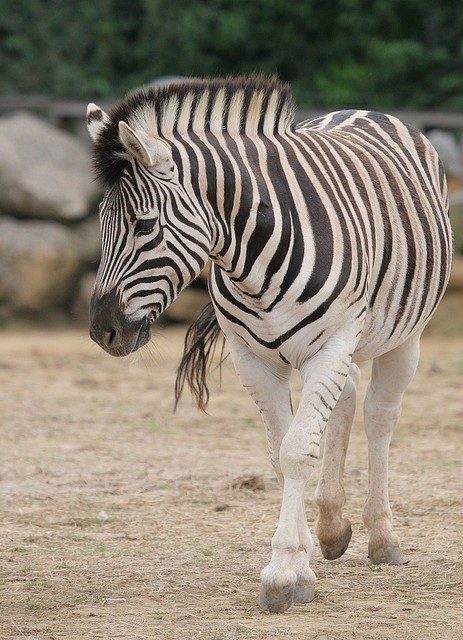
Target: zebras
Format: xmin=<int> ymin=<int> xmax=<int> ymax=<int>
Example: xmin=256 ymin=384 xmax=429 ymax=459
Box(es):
xmin=84 ymin=69 xmax=454 ymax=613
